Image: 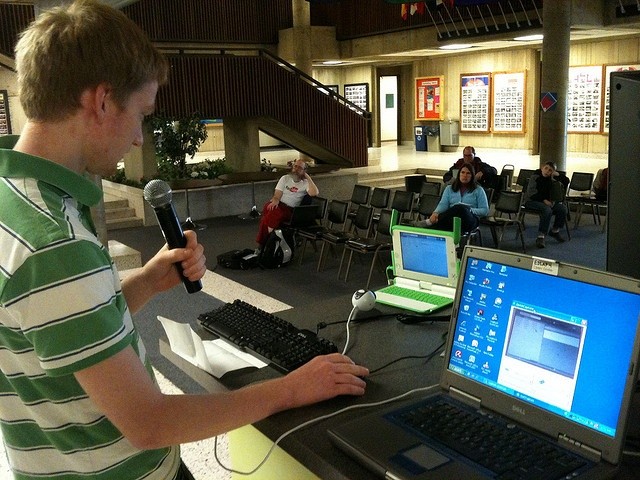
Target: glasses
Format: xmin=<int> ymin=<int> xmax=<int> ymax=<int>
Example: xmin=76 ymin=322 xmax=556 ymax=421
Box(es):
xmin=293 ymin=164 xmax=305 ymax=170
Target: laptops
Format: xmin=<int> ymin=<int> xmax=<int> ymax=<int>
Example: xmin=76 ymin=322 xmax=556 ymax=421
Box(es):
xmin=327 ymin=246 xmax=640 ymax=479
xmin=374 ymin=209 xmax=461 ymax=312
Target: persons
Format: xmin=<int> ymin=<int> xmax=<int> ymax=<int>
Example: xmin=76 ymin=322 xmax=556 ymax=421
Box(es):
xmin=255 ymin=159 xmax=319 ymax=256
xmin=522 ymin=161 xmax=568 ymax=248
xmin=443 ymin=146 xmax=498 ymax=188
xmin=1 ymin=2 xmax=370 ymax=480
xmin=414 ymin=163 xmax=490 ymax=236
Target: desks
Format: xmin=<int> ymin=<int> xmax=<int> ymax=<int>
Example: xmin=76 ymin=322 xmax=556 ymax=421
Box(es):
xmin=158 ymin=278 xmax=491 ymax=480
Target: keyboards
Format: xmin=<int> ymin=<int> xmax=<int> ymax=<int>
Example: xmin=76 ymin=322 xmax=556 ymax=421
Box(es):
xmin=198 ymin=299 xmax=337 ymax=374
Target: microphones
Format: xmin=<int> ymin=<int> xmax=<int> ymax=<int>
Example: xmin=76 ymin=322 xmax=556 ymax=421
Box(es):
xmin=143 ymin=180 xmax=202 ymax=294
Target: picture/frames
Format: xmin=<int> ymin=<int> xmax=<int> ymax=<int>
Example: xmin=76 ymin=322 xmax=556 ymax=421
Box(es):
xmin=459 ymin=72 xmax=491 ymax=134
xmin=313 ymin=84 xmax=339 ymax=103
xmin=0 ymin=89 xmax=12 ymax=135
xmin=603 ymin=62 xmax=640 ymax=135
xmin=565 ymin=64 xmax=604 ymax=135
xmin=490 ymin=68 xmax=527 ymax=135
xmin=344 ymin=83 xmax=369 ymax=117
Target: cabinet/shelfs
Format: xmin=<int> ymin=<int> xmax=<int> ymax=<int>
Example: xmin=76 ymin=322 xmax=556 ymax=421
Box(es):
xmin=414 ymin=75 xmax=444 ymax=121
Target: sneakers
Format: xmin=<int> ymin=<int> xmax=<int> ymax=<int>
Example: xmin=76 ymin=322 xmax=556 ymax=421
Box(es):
xmin=415 ymin=220 xmax=432 ymax=228
xmin=548 ymin=228 xmax=565 ymax=243
xmin=536 ymin=237 xmax=546 ymax=249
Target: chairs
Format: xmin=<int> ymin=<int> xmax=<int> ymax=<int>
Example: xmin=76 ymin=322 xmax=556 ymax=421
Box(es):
xmin=411 ymin=181 xmax=441 ymax=222
xmin=457 ymin=188 xmax=494 ymax=248
xmin=319 ymin=204 xmax=375 ymax=280
xmin=478 ymin=190 xmax=527 ymax=253
xmin=350 ymin=187 xmax=391 ymax=240
xmin=491 ymin=175 xmax=511 ymax=219
xmin=391 ymin=190 xmax=416 ymax=225
xmin=510 ymin=168 xmax=534 ymax=193
xmin=515 ymin=177 xmax=572 ymax=242
xmin=296 ymin=198 xmax=349 ymax=273
xmin=278 ymin=195 xmax=327 ymax=267
xmin=344 ymin=208 xmax=400 ymax=289
xmin=273 ymin=192 xmax=315 ymax=253
xmin=573 ymin=169 xmax=609 ymax=233
xmin=335 ymin=184 xmax=370 ymax=236
xmin=564 ymin=172 xmax=596 ymax=230
xmin=402 ymin=193 xmax=441 ymax=227
xmin=500 ymin=164 xmax=515 ymax=191
xmin=402 ymin=174 xmax=425 ymax=223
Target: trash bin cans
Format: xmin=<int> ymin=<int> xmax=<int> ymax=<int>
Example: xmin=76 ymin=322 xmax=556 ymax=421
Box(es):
xmin=414 ymin=126 xmax=427 ymax=151
xmin=425 ymin=125 xmax=442 ymax=152
